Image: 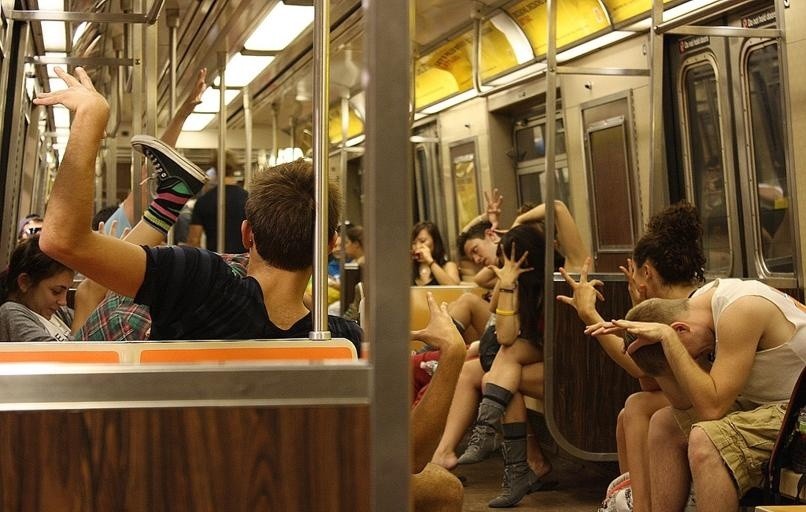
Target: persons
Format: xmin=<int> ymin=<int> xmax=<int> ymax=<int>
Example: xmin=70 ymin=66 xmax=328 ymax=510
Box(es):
xmin=1 ymin=63 xmax=366 ymax=354
xmin=411 ymin=189 xmax=806 ymax=512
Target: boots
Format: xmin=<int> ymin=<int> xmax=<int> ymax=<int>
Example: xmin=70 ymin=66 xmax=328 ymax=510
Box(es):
xmin=488 ymin=438 xmax=553 ymax=508
xmin=457 ymin=398 xmax=505 ymax=464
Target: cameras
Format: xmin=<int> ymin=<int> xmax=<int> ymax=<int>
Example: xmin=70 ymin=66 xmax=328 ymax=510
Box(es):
xmin=411 ymin=249 xmax=420 ymax=261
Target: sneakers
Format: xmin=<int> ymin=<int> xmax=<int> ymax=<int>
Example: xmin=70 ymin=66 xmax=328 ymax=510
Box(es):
xmin=131 ymin=135 xmax=210 ymax=198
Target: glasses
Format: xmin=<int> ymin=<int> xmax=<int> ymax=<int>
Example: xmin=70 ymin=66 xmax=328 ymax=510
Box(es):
xmin=28 ymin=227 xmax=42 ymax=235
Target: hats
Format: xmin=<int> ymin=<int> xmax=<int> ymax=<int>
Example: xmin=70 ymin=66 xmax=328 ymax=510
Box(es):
xmin=17 ymin=213 xmax=44 ymax=239
